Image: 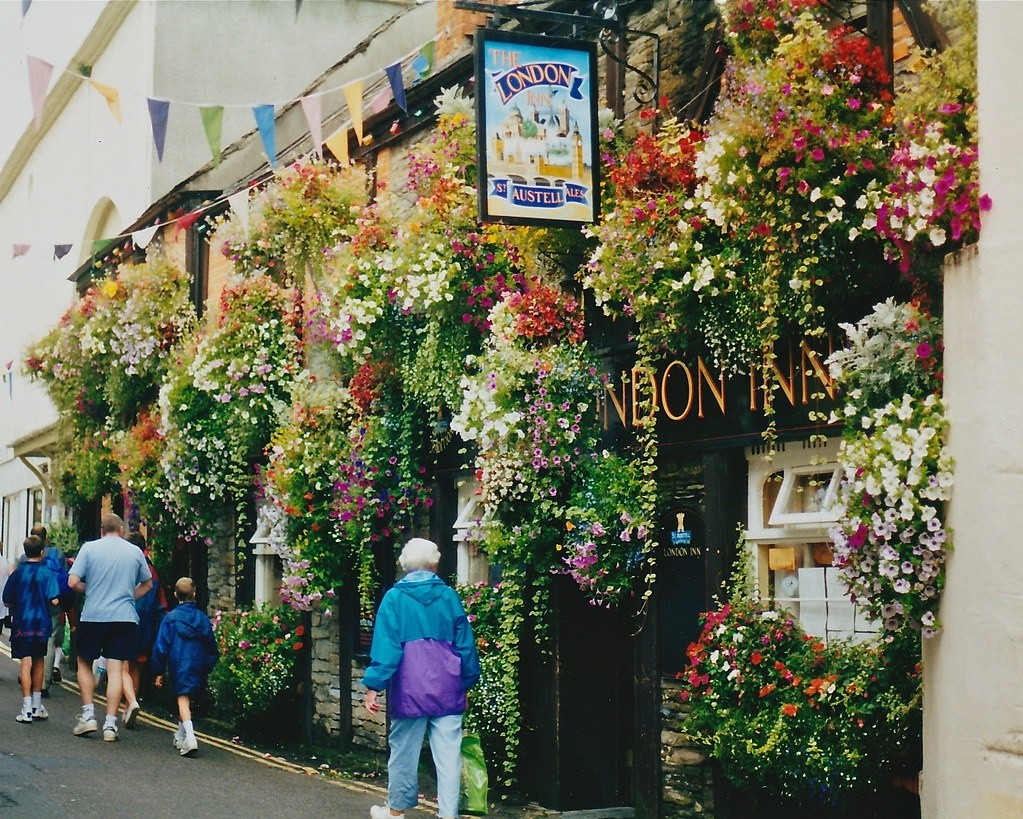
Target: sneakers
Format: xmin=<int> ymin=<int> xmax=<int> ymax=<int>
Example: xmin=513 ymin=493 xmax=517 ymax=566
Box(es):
xmin=172 ymin=734 xmax=184 ymax=749
xmin=73 ymin=714 xmax=98 ymax=735
xmin=102 ymin=725 xmax=119 ymax=741
xmin=180 ymin=738 xmax=198 ymax=756
xmin=16 ymin=708 xmax=32 ymax=722
xmin=32 ymin=705 xmax=49 ymax=718
xmin=370 ymin=801 xmax=405 ymax=819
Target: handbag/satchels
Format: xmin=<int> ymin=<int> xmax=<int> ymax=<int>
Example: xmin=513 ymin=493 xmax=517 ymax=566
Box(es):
xmin=457 ymin=710 xmax=489 ymax=816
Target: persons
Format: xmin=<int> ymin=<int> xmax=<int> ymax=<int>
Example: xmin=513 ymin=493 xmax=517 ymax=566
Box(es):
xmin=67 ymin=513 xmax=165 ymax=743
xmin=0 ymin=526 xmax=67 ymax=722
xmin=363 ymin=538 xmax=480 ymax=819
xmin=152 ymin=577 xmax=218 ymax=755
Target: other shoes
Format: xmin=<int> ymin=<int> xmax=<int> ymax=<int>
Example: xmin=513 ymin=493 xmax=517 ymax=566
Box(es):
xmin=53 ymin=667 xmax=62 ymax=682
xmin=41 ymin=689 xmax=49 ymax=698
xmin=121 ymin=703 xmax=141 ymax=729
